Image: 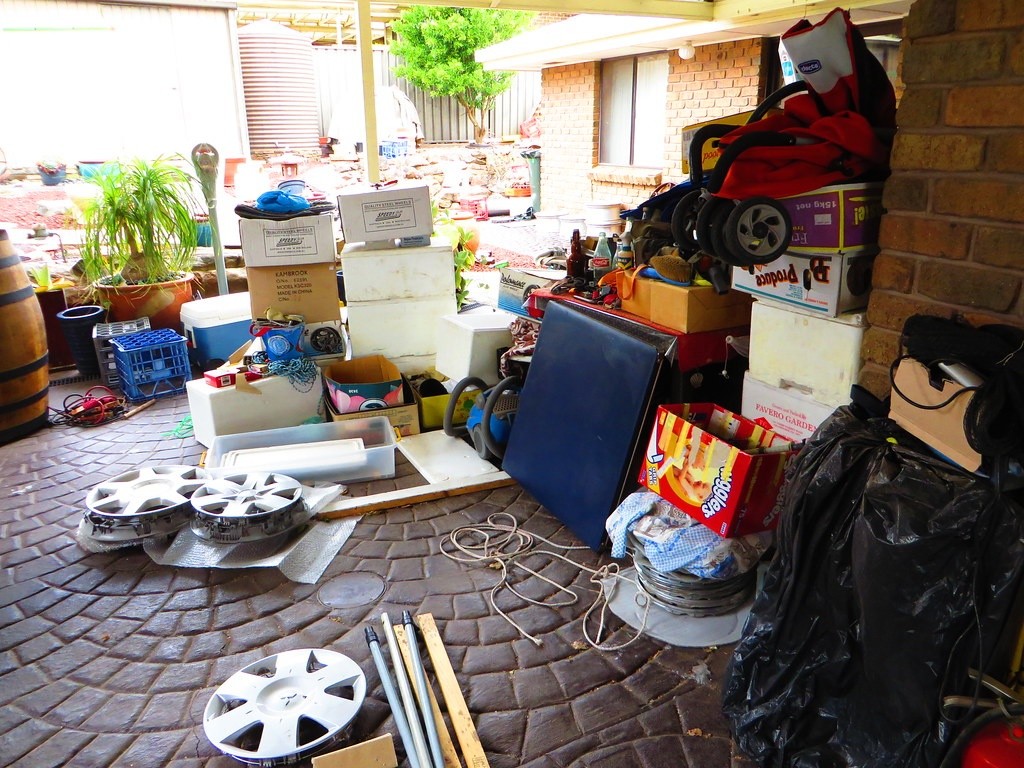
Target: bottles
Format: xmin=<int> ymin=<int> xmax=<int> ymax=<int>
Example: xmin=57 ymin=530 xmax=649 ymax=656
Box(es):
xmin=592 ymin=232 xmax=610 ymax=280
xmin=566 ymin=229 xmax=587 ymax=277
xmin=613 ymin=233 xmax=635 ymax=271
xmin=641 ymin=206 xmax=661 ymax=224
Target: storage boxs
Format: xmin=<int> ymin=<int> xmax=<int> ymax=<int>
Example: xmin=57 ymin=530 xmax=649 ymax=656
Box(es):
xmin=87 ymin=106 xmax=882 ymax=539
xmin=888 ymin=355 xmax=989 ymax=472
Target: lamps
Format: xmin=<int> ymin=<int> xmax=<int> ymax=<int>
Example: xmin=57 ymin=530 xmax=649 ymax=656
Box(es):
xmin=678 ymin=39 xmax=697 ymax=59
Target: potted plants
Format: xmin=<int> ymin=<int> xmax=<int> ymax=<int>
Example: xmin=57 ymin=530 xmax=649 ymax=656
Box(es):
xmin=88 ymin=154 xmax=206 ymax=331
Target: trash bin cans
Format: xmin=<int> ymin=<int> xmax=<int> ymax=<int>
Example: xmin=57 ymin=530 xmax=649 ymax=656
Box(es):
xmin=520 ymin=150 xmax=540 ymax=212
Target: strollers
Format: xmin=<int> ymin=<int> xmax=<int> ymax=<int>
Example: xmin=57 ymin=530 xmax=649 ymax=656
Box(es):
xmin=669 ymin=7 xmax=900 ymax=274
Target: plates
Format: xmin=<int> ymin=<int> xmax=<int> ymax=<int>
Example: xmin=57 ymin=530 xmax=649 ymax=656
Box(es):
xmin=625 ymin=530 xmax=773 ymax=618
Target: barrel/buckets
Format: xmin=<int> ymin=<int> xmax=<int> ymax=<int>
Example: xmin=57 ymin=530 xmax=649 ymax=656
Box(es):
xmin=0 ymin=229 xmax=50 ymax=446
